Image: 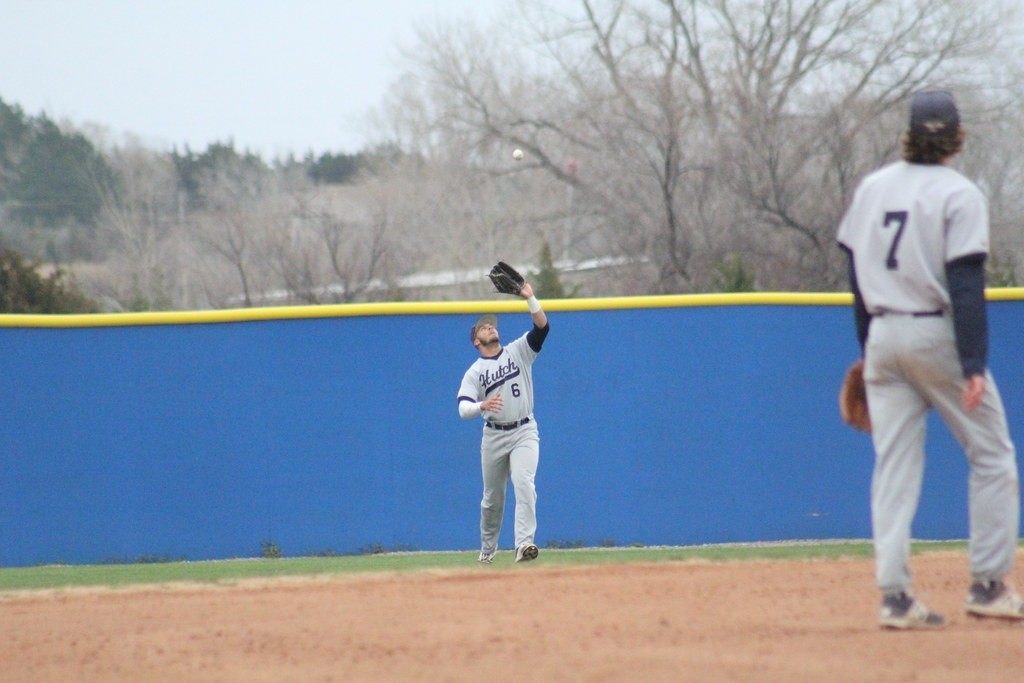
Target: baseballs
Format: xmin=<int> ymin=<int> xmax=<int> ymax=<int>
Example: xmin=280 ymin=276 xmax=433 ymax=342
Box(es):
xmin=513 ymin=149 xmax=523 ymax=160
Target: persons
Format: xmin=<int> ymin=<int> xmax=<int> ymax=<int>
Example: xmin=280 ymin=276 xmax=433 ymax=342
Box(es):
xmin=834 ymin=86 xmax=1024 ymax=633
xmin=456 ymin=262 xmax=549 ymax=562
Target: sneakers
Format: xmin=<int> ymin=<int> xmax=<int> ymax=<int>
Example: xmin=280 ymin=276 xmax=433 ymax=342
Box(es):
xmin=479 ymin=544 xmax=498 ymax=563
xmin=515 ymin=544 xmax=538 ymax=563
xmin=965 ymin=581 xmax=1023 ymax=618
xmin=878 ymin=592 xmax=947 ymax=629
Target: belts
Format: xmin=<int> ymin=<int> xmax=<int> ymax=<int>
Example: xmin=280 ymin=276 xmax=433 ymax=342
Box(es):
xmin=866 ymin=310 xmax=943 ymax=317
xmin=486 ymin=416 xmax=529 ymax=431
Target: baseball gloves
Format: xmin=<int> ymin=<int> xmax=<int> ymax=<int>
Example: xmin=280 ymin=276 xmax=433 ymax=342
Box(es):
xmin=839 ymin=359 xmax=871 ymax=434
xmin=490 ymin=260 xmax=527 ymax=295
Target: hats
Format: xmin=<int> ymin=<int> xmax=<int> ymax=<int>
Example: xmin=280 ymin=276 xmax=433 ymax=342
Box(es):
xmin=470 ymin=314 xmax=497 ymax=342
xmin=910 ymin=90 xmax=959 ymax=126
